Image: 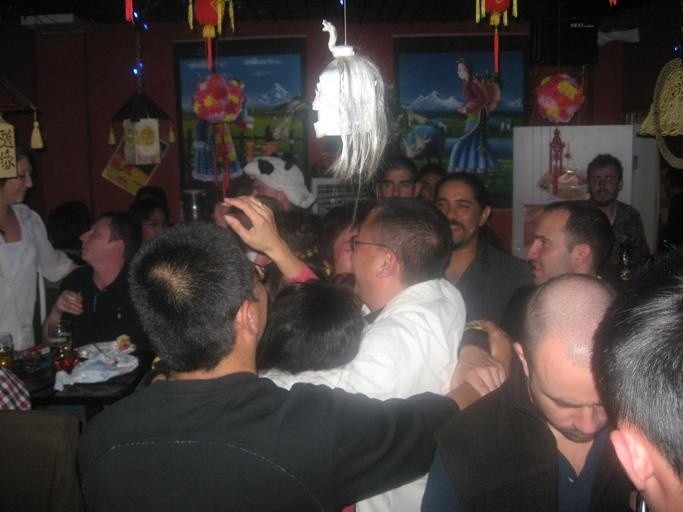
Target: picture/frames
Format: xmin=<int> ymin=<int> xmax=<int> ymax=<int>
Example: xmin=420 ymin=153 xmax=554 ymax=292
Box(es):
xmin=175 ymin=38 xmax=306 ymax=208
xmin=395 ymin=36 xmax=529 ymax=208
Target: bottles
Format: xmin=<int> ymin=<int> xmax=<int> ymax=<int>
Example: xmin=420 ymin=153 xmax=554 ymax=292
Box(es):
xmin=615 ymin=239 xmax=635 ymax=282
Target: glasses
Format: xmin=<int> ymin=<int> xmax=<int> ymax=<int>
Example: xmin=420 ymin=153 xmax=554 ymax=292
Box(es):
xmin=349 ymin=235 xmax=399 ymax=262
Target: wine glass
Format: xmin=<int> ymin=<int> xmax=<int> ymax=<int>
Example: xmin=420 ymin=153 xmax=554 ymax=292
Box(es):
xmin=0 ymin=317 xmax=72 ymax=375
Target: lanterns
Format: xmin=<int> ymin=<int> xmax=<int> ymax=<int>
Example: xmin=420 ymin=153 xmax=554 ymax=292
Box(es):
xmin=188 ymin=0 xmax=236 ymax=69
xmin=476 ymin=0 xmax=518 ymax=71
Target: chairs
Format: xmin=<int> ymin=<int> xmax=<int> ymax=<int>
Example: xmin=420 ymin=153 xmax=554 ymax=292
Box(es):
xmin=0 ymin=410 xmax=85 ymax=512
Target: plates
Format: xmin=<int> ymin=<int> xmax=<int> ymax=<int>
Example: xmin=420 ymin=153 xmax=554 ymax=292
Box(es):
xmin=112 ymin=354 xmax=140 ymax=377
xmin=75 ymin=340 xmax=138 ymax=360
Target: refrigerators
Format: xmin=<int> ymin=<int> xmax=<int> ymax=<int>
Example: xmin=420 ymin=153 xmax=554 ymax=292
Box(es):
xmin=508 ymin=122 xmax=663 ymax=263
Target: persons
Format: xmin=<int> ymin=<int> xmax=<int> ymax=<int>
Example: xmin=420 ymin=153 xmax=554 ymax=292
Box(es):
xmin=448 ymin=57 xmax=501 ymax=172
xmin=0 ymin=148 xmax=79 ymax=347
xmin=2 ymin=156 xmax=682 ymax=512
xmin=313 ymin=55 xmax=387 ymax=178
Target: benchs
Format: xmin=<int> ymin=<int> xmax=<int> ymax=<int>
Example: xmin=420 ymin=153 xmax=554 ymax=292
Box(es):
xmin=44 ymin=276 xmax=64 ymax=318
